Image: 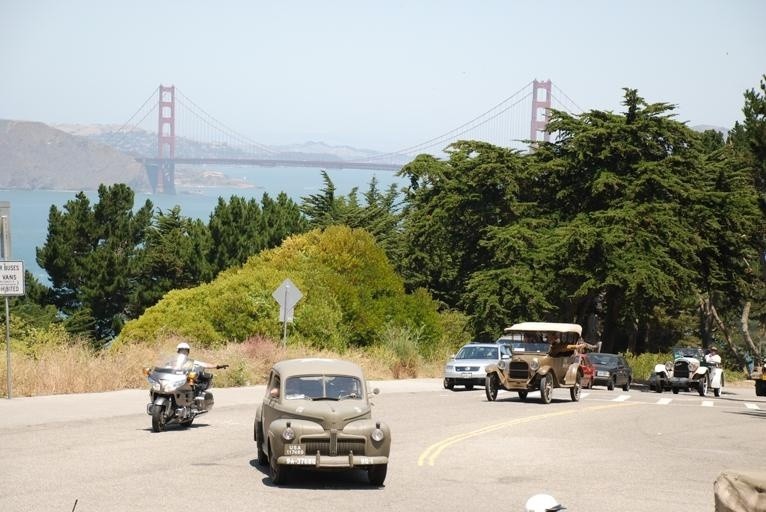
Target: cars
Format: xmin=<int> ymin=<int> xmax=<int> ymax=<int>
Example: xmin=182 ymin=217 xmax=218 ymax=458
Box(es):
xmin=252 ymin=359 xmax=391 ymax=490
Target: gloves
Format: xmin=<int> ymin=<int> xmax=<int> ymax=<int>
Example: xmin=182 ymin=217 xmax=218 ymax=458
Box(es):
xmin=216 ymin=364 xmax=229 ymax=370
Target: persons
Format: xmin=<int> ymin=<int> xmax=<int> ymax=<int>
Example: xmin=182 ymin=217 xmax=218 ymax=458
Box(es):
xmin=337 ymin=379 xmax=359 ymax=398
xmin=703 ymin=345 xmax=722 ymax=369
xmin=270 ymin=377 xmax=300 ymax=397
xmin=543 ymin=331 xmax=587 ymax=357
xmin=167 ymin=341 xmax=231 ymax=415
xmin=515 ymin=331 xmax=537 ymax=350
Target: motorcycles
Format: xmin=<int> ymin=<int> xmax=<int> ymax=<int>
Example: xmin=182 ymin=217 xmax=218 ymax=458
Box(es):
xmin=141 ymin=354 xmax=215 ymax=432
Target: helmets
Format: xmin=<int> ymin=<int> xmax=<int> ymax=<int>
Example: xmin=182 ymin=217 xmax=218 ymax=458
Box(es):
xmin=177 ymin=342 xmax=190 ymax=353
